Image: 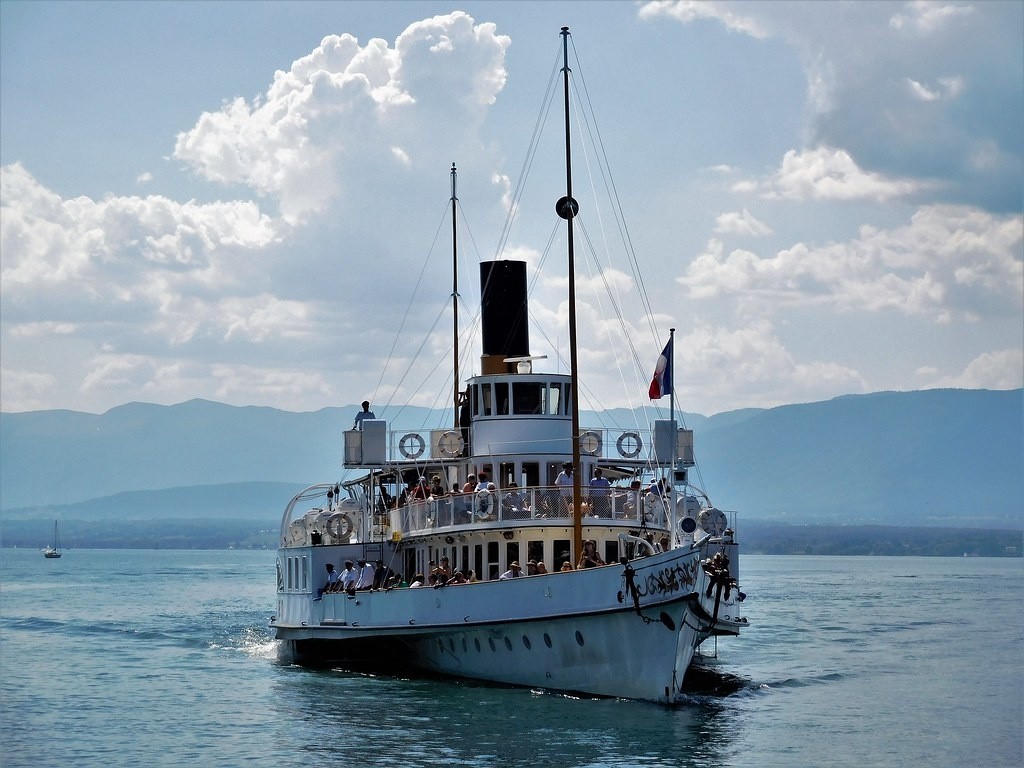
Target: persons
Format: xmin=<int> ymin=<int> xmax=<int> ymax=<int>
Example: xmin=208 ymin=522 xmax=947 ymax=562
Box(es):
xmin=351 ymin=401 xmax=376 ymax=431
xmin=312 ymin=460 xmax=672 ymax=601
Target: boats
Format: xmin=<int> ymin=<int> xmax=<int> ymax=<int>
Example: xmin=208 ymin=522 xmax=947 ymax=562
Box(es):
xmin=269 ymin=26 xmax=751 ymax=709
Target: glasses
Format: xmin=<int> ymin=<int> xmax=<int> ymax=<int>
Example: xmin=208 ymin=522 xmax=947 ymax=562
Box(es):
xmin=564 ymin=566 xmax=570 ymax=568
xmin=586 ymin=542 xmax=593 ymax=547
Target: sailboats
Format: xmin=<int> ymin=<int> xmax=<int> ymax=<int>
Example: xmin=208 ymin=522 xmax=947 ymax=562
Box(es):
xmin=44 ymin=519 xmax=62 ymax=558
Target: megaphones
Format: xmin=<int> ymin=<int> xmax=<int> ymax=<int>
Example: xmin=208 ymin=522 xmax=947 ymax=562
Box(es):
xmin=555 ymin=196 xmax=579 ymax=220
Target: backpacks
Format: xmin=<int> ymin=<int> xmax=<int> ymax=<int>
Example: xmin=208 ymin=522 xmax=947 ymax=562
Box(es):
xmin=471 ymin=482 xmax=480 ymax=502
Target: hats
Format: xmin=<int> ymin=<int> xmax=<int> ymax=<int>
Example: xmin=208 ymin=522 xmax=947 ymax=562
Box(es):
xmin=526 ymin=562 xmax=536 ymax=566
xmin=362 ymin=401 xmax=370 ymax=406
xmin=562 ymin=461 xmax=574 ymax=469
xmin=357 ymin=558 xmax=366 ymax=565
xmin=510 ymin=561 xmax=521 ymax=568
xmin=326 ymin=564 xmax=333 ymax=570
xmin=418 ymin=476 xmax=427 ymax=482
xmin=467 ymin=570 xmax=472 ymax=575
xmin=585 ymin=539 xmax=595 ymax=547
xmin=487 ymin=483 xmax=496 ymax=490
xmin=432 ymin=476 xmax=441 ymax=481
xmin=344 ymin=560 xmax=354 ymax=567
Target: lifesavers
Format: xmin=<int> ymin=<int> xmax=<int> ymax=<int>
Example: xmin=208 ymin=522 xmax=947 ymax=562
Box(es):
xmin=425 ymin=496 xmax=436 ymax=524
xmin=398 ymin=432 xmax=425 ymax=459
xmin=437 ymin=430 xmax=465 ymax=457
xmin=326 ymin=512 xmax=353 ymax=539
xmin=701 ymin=507 xmax=728 ymax=536
xmin=579 ymin=431 xmax=602 ymax=455
xmin=475 ymin=488 xmax=494 ymax=519
xmin=616 ymin=431 xmax=643 ymax=458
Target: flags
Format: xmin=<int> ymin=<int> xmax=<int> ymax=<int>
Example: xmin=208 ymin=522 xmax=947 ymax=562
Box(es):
xmin=648 ymin=336 xmax=672 ymax=400
